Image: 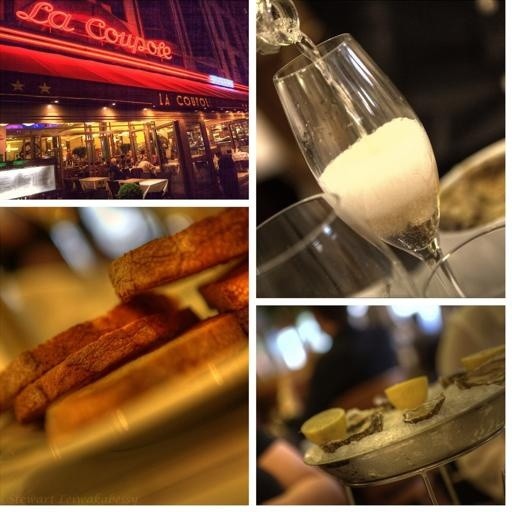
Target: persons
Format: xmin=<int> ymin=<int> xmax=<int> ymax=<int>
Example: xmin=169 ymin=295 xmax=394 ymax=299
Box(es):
xmin=227 ymin=149 xmax=247 ymax=173
xmin=101 ymin=157 xmax=108 ymax=165
xmin=257 ymin=417 xmax=350 ymax=504
xmin=304 ymin=305 xmax=405 ymax=417
xmin=212 ymin=150 xmax=240 ymax=199
xmin=137 ymin=156 xmax=157 ymax=179
xmin=109 ymin=158 xmax=129 ymax=181
xmin=231 ymin=145 xmax=241 ymax=153
xmin=410 ymin=312 xmax=437 ymax=376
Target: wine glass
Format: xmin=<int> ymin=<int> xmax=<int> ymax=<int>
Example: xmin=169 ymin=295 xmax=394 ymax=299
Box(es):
xmin=258 ymin=32 xmax=504 ymax=298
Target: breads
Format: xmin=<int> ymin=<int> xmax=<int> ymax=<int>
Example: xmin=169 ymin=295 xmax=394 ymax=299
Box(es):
xmin=1 ymin=208 xmax=248 ymax=438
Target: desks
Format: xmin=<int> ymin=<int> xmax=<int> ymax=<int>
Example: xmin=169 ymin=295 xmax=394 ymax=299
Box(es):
xmin=118 ymin=178 xmax=169 ymax=199
xmin=79 ymin=176 xmax=110 ymax=199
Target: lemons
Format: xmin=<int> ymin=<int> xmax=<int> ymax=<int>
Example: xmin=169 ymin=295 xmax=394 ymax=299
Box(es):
xmin=383 ymin=375 xmax=429 ymax=411
xmin=299 ymin=407 xmax=348 ymax=446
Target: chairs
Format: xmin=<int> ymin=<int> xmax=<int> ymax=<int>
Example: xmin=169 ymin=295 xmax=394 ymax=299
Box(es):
xmin=121 ymin=167 xmax=145 ymax=178
